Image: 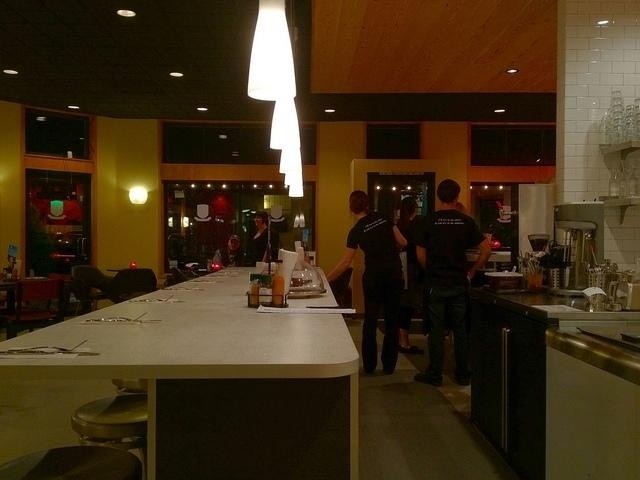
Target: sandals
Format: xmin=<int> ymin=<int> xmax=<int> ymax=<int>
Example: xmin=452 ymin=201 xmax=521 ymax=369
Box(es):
xmin=398 ymin=344 xmax=424 ymax=354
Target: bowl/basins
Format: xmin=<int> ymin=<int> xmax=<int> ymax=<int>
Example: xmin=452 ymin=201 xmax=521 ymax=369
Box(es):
xmin=484 ymin=272 xmax=522 ymax=289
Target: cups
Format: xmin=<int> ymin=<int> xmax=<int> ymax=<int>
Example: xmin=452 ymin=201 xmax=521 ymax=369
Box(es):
xmin=606 ymin=90 xmax=640 ymax=140
xmin=129 ymin=256 xmax=137 ymax=269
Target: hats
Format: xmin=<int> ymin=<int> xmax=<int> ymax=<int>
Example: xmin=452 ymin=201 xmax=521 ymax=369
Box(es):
xmin=230 ymin=235 xmax=241 ymax=241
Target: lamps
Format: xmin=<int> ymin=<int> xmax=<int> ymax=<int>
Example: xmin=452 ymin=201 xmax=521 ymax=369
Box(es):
xmin=247 ymin=0 xmax=303 ymax=199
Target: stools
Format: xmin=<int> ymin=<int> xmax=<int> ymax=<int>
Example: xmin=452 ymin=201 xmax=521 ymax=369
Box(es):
xmin=0 ymin=376 xmax=148 ymax=480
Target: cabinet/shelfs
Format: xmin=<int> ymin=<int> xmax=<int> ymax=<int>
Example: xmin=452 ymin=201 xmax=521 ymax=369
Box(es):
xmin=470 ymin=298 xmax=545 ymax=480
xmin=599 ymin=141 xmax=640 ymax=208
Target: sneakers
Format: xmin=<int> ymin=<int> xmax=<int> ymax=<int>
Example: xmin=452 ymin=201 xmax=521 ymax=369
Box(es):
xmin=414 ymin=373 xmax=442 ymax=386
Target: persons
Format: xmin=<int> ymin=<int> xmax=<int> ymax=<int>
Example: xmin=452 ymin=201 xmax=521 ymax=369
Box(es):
xmin=326 ymin=178 xmax=491 ymax=387
xmin=213 ymin=211 xmax=279 ymax=266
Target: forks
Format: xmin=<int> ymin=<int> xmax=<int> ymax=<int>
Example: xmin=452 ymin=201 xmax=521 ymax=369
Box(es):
xmin=9 ymin=339 xmax=89 ymax=351
xmin=139 ymin=294 xmax=174 ymax=302
xmin=86 ymin=312 xmax=148 ymax=322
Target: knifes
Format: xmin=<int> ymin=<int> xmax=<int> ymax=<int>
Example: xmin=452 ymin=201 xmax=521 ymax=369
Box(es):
xmin=0 ymin=350 xmax=100 ymax=357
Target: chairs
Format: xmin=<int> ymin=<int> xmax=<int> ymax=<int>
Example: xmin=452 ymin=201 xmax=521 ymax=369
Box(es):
xmin=0 ymin=264 xmax=210 ymax=340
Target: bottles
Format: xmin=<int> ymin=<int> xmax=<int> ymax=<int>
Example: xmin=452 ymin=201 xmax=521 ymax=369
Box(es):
xmin=610 ymin=159 xmax=640 ymax=197
xmin=2 ymin=264 xmax=12 ymax=282
